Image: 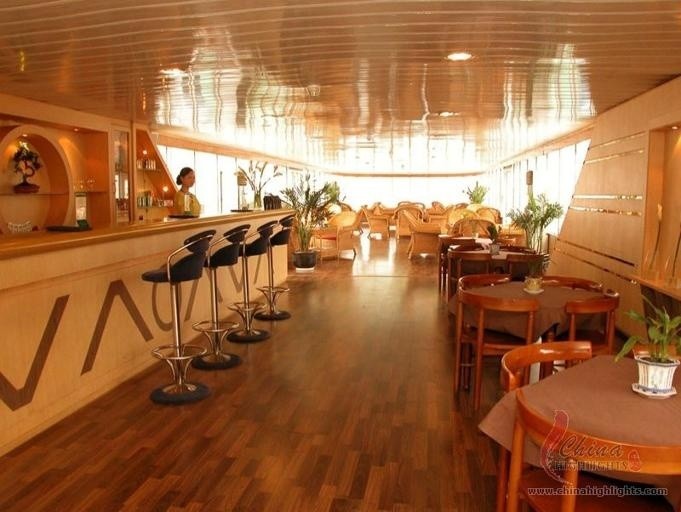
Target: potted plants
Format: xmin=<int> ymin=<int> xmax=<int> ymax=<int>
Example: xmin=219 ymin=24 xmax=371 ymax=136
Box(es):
xmin=239 ymin=160 xmax=283 ymax=208
xmin=280 ymin=167 xmax=341 ymax=268
xmin=8 ymin=141 xmax=44 ymax=193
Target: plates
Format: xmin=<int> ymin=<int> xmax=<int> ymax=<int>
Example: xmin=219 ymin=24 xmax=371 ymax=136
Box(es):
xmin=524 ymin=288 xmax=544 ymax=294
xmin=631 ymin=382 xmax=678 ymax=399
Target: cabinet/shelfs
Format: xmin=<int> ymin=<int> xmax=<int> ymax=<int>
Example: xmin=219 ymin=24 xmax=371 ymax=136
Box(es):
xmin=0 ymin=93 xmax=179 ymax=239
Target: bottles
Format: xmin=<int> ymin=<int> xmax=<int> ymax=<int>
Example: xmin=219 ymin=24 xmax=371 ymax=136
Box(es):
xmin=115 ymin=198 xmax=129 ymax=209
xmin=138 ymin=190 xmax=161 ymax=207
xmin=136 ymin=157 xmax=156 ymax=169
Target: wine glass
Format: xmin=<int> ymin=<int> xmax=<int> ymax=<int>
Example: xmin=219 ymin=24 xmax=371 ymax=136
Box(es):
xmin=74 ymin=175 xmax=95 ymax=190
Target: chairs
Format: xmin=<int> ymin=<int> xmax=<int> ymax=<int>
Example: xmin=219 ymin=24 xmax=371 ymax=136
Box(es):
xmin=254 ymin=215 xmax=294 ymax=320
xmin=190 ymin=224 xmax=251 ymax=370
xmin=226 ymin=220 xmax=278 ymax=343
xmin=142 ymin=230 xmax=217 ymax=404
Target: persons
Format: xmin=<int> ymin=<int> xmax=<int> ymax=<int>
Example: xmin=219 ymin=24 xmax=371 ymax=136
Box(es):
xmin=172 ymin=167 xmax=201 ymax=219
